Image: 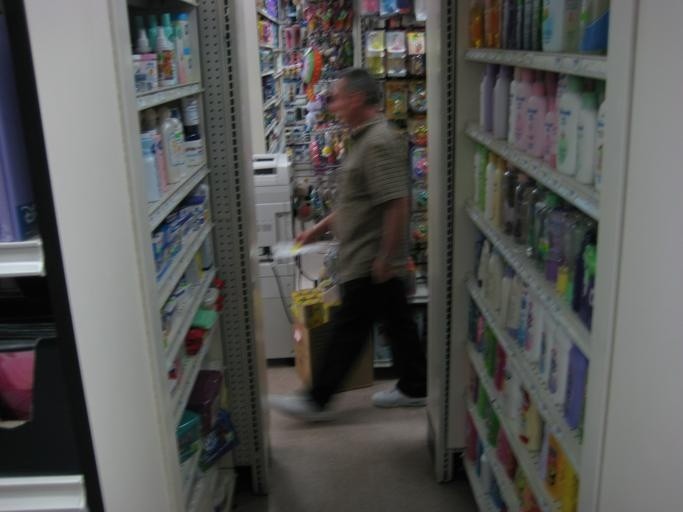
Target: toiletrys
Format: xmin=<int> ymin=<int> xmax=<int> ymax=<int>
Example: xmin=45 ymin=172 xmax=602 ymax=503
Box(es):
xmin=461 ymin=0 xmax=611 ymax=512
xmin=131 ymin=7 xmax=209 ymax=205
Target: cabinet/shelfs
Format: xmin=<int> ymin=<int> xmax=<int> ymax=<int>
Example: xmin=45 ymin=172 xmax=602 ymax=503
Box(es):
xmin=421 ymin=1 xmax=681 ymax=512
xmin=1 ymin=1 xmax=270 ymax=512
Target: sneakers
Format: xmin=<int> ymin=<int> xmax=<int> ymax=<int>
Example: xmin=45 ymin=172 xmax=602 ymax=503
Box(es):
xmin=372 ymin=387 xmax=426 ymax=407
xmin=268 ymin=393 xmax=336 ymax=422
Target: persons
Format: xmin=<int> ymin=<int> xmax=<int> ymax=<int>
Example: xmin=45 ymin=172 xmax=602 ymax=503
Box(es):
xmin=265 ymin=66 xmax=428 ymax=423
